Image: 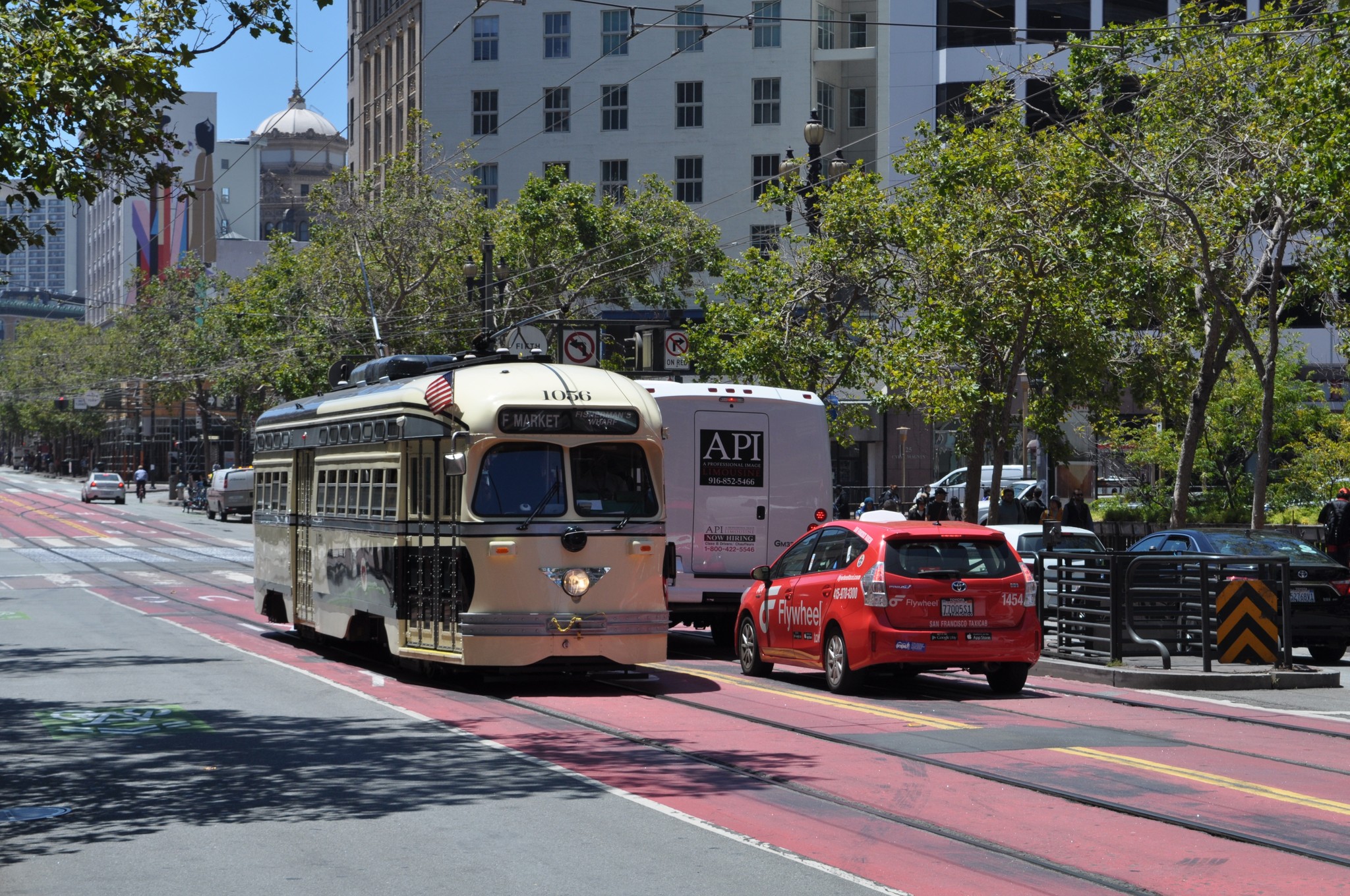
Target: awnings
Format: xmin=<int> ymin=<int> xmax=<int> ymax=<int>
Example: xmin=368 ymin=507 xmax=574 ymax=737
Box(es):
xmin=683 ymin=376 xmax=887 ymax=404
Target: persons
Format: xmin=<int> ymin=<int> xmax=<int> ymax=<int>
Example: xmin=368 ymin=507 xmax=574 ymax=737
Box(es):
xmin=882 ymin=485 xmax=901 ymax=512
xmin=1020 ymin=488 xmax=1047 ymax=524
xmin=908 ymin=484 xmax=931 ymax=521
xmin=834 ymin=485 xmax=850 ymax=520
xmin=1318 ymin=487 xmax=1350 ymax=568
xmin=213 ymin=461 xmax=221 ymax=472
xmin=1039 ymin=496 xmax=1063 ymax=525
xmin=134 ymin=465 xmax=148 ymax=498
xmin=856 ymin=497 xmax=875 ymax=520
xmin=1061 ymin=488 xmax=1094 ymax=532
xmin=578 ymin=454 xmax=628 ymax=501
xmin=0 ymin=447 xmax=60 ymax=473
xmin=947 ymin=496 xmax=963 ymax=521
xmin=997 ymin=487 xmax=1025 ymax=525
xmin=81 ymin=456 xmax=87 ymax=476
xmin=981 ymin=487 xmax=991 ymax=501
xmin=925 ymin=488 xmax=950 ymax=521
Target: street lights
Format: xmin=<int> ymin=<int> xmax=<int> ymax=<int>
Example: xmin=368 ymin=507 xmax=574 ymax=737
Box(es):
xmin=461 ymin=233 xmax=511 ymax=337
xmin=1018 ymin=372 xmax=1031 ymax=479
xmin=896 ymin=425 xmax=912 ymax=503
xmin=776 ymin=108 xmax=854 ymax=231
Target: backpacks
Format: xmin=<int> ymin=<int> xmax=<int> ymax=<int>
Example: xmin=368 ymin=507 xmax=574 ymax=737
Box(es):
xmin=878 ymin=490 xmax=891 ymax=507
xmin=1327 ymin=501 xmax=1350 ymax=542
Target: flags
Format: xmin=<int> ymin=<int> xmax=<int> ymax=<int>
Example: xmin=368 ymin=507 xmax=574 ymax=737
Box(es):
xmin=425 ymin=370 xmax=453 ymax=415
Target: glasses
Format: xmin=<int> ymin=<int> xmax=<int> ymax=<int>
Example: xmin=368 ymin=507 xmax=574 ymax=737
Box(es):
xmin=918 ymin=502 xmax=926 ymax=506
xmin=1074 ymin=493 xmax=1084 ymax=496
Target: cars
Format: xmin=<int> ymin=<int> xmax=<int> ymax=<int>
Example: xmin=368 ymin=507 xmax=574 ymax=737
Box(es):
xmin=1121 ymin=528 xmax=1350 ymax=661
xmin=79 ymin=471 xmax=127 ymax=505
xmin=733 ymin=521 xmax=1043 ymax=694
xmin=947 ymin=480 xmax=1036 ymax=526
xmin=957 ymin=524 xmax=1107 ymax=609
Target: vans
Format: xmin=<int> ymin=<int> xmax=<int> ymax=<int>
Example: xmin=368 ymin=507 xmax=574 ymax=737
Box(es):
xmin=913 ymin=464 xmax=1032 ymax=503
xmin=207 ymin=467 xmax=253 ymax=523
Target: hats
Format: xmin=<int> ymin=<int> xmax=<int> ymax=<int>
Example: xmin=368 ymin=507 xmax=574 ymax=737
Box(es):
xmin=934 ymin=488 xmax=948 ymax=495
xmin=1050 ymin=495 xmax=1060 ymax=501
xmin=864 ymin=497 xmax=874 ymax=504
xmin=860 ymin=502 xmax=865 ymax=506
xmin=834 ymin=485 xmax=842 ymax=489
xmin=1339 ymin=487 xmax=1350 ymax=495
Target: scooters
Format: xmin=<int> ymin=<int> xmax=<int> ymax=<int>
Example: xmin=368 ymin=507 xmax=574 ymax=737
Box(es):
xmin=138 ymin=482 xmax=145 ymax=502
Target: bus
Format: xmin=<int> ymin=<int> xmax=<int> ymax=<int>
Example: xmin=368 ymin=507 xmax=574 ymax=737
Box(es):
xmin=252 ymin=232 xmax=677 ymax=670
xmin=634 ymin=378 xmax=835 ymax=654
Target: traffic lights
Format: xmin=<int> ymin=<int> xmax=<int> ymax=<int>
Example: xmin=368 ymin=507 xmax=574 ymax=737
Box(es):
xmin=622 ymin=333 xmax=642 ymax=371
